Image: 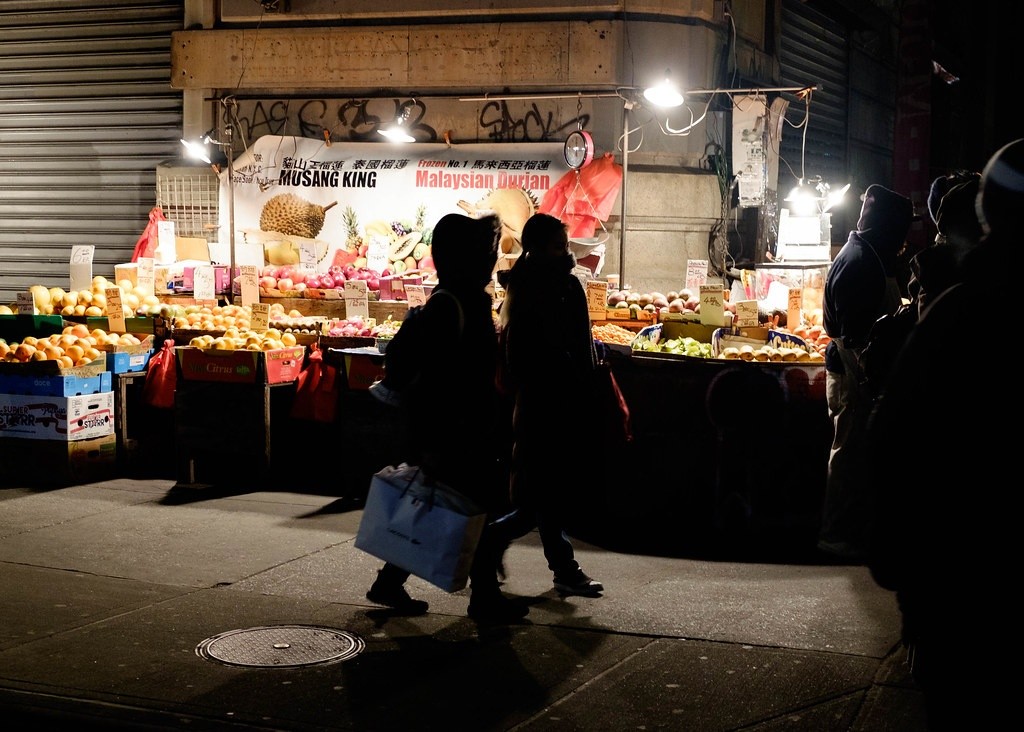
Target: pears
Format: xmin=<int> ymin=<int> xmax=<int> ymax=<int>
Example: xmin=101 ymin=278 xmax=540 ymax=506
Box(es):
xmin=189 ymin=327 xmax=296 ymax=350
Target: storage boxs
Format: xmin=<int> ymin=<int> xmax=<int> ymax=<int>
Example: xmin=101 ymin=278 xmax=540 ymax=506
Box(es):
xmin=0 ymin=350 xmax=117 ymax=487
xmin=156 ymin=159 xmax=221 ymax=243
xmin=91 ymin=331 xmax=156 ymax=374
xmin=176 ymin=345 xmax=307 ymax=384
xmin=662 ymin=321 xmax=769 ymax=343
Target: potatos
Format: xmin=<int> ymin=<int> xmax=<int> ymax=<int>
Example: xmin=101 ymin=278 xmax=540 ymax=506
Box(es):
xmin=723 ymin=345 xmax=825 ymax=362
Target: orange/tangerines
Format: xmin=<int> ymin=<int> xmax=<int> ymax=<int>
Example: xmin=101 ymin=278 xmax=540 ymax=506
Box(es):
xmin=0 ymin=324 xmax=143 ymax=368
xmin=0 ymin=276 xmax=160 ymax=318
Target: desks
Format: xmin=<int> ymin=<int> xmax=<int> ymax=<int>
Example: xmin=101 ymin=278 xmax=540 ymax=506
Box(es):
xmin=189 ymin=381 xmax=295 ymax=487
xmin=117 ymin=371 xmax=150 ymax=450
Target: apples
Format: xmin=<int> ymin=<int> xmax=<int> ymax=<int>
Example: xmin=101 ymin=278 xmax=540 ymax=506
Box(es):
xmin=392 ymin=255 xmax=435 ymax=273
xmin=609 ymin=289 xmax=736 ymax=319
xmin=258 ymin=262 xmax=393 ymax=292
xmin=792 ymin=327 xmax=832 ymax=357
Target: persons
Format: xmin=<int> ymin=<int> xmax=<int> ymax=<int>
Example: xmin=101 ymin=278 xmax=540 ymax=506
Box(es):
xmin=821 ymin=139 xmax=1024 ymax=732
xmin=365 ymin=213 xmax=611 ymax=618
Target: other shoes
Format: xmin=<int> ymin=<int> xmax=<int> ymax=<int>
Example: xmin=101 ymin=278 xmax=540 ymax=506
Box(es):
xmin=549 ymin=573 xmax=604 ymax=599
xmin=482 ymin=525 xmax=507 ymax=583
xmin=365 ymin=580 xmax=429 ymax=617
xmin=467 ymin=594 xmax=529 ymax=622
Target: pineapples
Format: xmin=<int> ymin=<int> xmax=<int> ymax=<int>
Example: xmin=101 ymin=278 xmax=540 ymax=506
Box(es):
xmin=341 ymin=204 xmax=362 ymax=252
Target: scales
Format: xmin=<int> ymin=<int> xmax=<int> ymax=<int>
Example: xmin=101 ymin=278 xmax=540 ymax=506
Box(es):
xmin=556 ymin=97 xmax=610 ymax=260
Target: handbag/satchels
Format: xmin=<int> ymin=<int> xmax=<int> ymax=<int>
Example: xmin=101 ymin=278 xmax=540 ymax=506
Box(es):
xmin=368 ymin=290 xmax=464 ymax=404
xmin=354 ymin=463 xmax=487 ymax=594
xmin=591 ymin=345 xmax=633 ymax=433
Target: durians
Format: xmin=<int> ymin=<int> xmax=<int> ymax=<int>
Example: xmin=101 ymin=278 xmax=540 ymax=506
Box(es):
xmin=456 ymin=185 xmax=540 ymax=258
xmin=241 ymin=192 xmax=338 ymax=268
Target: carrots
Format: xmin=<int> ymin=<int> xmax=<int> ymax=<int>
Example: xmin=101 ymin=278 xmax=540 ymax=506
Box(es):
xmin=768 ymin=313 xmax=779 ymax=326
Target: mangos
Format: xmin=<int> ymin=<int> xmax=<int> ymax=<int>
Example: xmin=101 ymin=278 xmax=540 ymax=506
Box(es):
xmin=134 ymin=302 xmax=304 ymax=331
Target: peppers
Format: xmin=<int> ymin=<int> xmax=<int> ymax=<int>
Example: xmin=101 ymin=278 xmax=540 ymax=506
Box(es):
xmin=633 ymin=335 xmax=711 ymax=359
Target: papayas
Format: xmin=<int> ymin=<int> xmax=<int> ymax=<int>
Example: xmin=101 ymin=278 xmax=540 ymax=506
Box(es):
xmin=388 ymin=231 xmax=422 ymax=261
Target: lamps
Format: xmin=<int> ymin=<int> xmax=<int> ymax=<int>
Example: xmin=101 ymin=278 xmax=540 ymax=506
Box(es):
xmin=784 ymin=178 xmax=824 ymax=203
xmin=180 ymin=127 xmax=233 ymax=165
xmin=815 ymin=180 xmax=851 ymax=212
xmin=377 ymin=98 xmax=417 ymax=143
xmin=643 ymin=68 xmax=684 ymax=108
